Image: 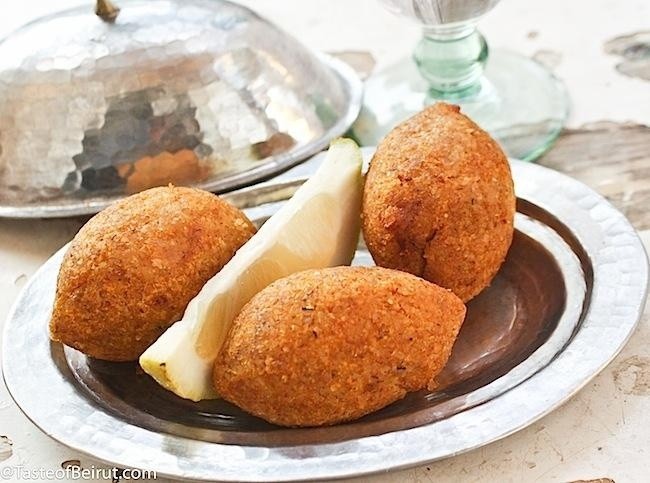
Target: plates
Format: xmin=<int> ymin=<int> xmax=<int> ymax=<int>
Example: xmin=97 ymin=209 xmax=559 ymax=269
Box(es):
xmin=1 ymin=143 xmax=650 ymax=483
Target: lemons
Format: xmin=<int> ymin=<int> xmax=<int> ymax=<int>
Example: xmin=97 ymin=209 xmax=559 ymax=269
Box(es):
xmin=139 ymin=135 xmax=362 ymax=402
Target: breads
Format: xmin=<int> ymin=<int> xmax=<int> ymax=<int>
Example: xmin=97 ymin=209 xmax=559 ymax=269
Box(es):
xmin=361 ymin=102 xmax=515 ymax=304
xmin=48 ymin=183 xmax=257 ymax=363
xmin=211 ymin=266 xmax=467 ymax=427
